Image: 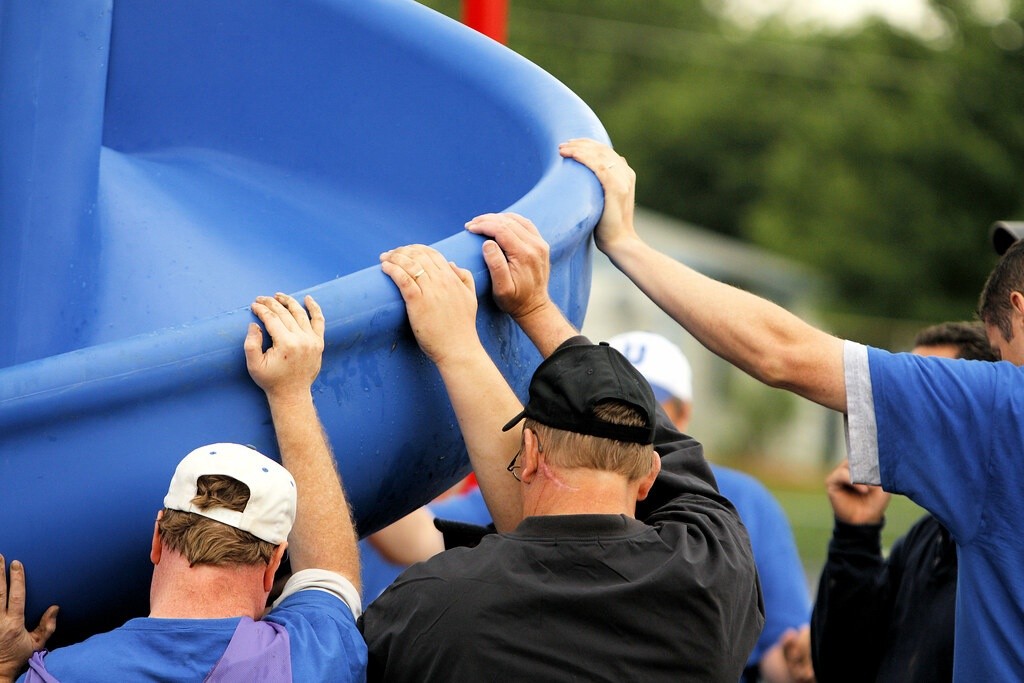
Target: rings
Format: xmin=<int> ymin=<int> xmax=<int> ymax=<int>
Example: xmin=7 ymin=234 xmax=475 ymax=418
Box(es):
xmin=413 ymin=270 xmax=424 ymax=280
xmin=608 ymin=162 xmax=615 ymax=167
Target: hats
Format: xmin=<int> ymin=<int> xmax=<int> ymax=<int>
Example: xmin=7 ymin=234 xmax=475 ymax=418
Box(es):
xmin=163 ymin=443 xmax=297 ymax=545
xmin=502 ymin=341 xmax=655 ymax=445
xmin=601 ymin=331 xmax=691 ymax=405
xmin=992 ymin=221 xmax=1024 ymax=256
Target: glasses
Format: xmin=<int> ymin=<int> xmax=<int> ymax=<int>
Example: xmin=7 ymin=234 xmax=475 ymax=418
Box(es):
xmin=507 ymin=430 xmax=543 ymax=482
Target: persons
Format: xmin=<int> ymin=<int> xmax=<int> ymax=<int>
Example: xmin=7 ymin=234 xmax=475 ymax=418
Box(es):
xmin=809 ymin=322 xmax=1002 ymax=683
xmin=355 ymin=210 xmax=764 ymax=683
xmin=608 ymin=330 xmax=815 ymax=682
xmin=557 ymin=138 xmax=1024 ymax=683
xmin=0 ymin=291 xmax=368 ymax=683
